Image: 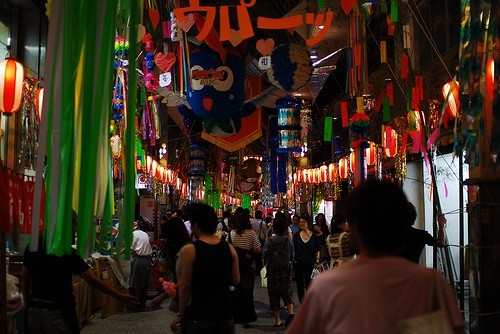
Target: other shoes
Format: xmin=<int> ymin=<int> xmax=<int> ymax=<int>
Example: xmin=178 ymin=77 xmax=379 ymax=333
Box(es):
xmin=288 ymin=314 xmax=294 ymax=319
xmin=274 ymin=321 xmax=283 ymax=327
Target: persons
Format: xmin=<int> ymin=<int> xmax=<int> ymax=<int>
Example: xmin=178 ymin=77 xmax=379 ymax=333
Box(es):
xmin=128 ymin=205 xmax=197 ymax=313
xmin=19 ymin=208 xmax=147 ymax=334
xmin=261 ymin=210 xmax=357 ymax=327
xmin=215 ymin=205 xmax=273 ymax=328
xmin=171 ymin=203 xmax=241 ymax=334
xmin=404 ymin=201 xmax=438 ymax=267
xmin=284 ymin=177 xmax=467 ymax=334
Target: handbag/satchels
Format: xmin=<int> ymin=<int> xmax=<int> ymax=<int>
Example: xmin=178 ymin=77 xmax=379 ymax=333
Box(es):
xmin=228 ymin=231 xmax=255 ymax=271
xmin=258 ymin=238 xmax=264 ymax=247
xmin=400 ymin=268 xmax=453 ymax=334
xmin=260 ymin=266 xmax=267 ymax=288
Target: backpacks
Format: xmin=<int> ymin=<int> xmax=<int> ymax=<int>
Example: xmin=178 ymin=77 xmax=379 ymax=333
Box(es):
xmin=268 ymin=236 xmax=291 ymax=279
